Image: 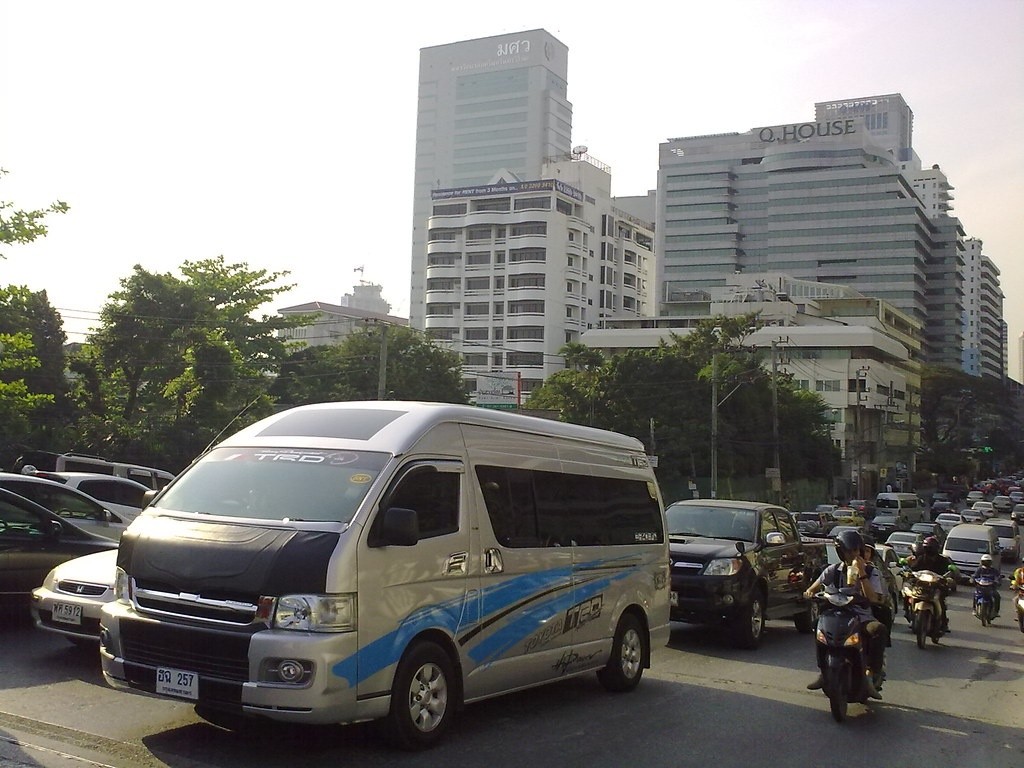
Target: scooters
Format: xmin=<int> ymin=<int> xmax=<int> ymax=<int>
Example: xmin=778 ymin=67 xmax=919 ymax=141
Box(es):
xmin=1009 ymin=575 xmax=1024 ymax=634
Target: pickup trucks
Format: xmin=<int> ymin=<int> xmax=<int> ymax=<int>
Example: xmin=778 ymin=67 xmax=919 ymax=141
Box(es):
xmin=663 ymin=498 xmax=832 ymax=649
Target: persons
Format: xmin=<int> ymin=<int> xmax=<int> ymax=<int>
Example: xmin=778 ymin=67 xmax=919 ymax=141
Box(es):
xmin=969 ymin=554 xmax=1001 ymax=618
xmin=905 ymin=537 xmax=962 ymax=633
xmin=1011 ymin=557 xmax=1024 ymax=622
xmin=886 ymin=478 xmax=912 ymax=493
xmin=802 ymin=529 xmax=894 ymax=700
xmin=790 ymin=545 xmax=822 ymax=570
xmin=829 ymin=495 xmax=858 ymax=505
xmin=782 ymin=495 xmax=792 ymax=511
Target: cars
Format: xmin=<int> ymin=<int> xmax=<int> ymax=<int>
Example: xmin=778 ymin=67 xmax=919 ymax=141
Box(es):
xmin=0 ymin=470 xmax=133 ymax=544
xmin=935 ymin=512 xmax=969 ymax=533
xmin=983 ymin=518 xmax=1021 ymax=563
xmin=929 ymin=470 xmax=1024 ymax=515
xmin=0 ymin=487 xmax=119 ymax=620
xmin=26 ymin=547 xmax=117 ymax=649
xmin=1011 ymin=504 xmax=1024 ymax=526
xmin=872 ymin=542 xmax=900 ymax=567
xmin=790 ymin=499 xmax=906 ymax=540
xmin=884 ymin=541 xmax=918 ymax=562
xmin=910 ymin=523 xmax=947 ymax=545
xmin=32 ymin=471 xmax=154 ymax=523
xmin=959 ymin=509 xmax=986 ymax=526
xmin=884 ymin=532 xmax=923 ymax=546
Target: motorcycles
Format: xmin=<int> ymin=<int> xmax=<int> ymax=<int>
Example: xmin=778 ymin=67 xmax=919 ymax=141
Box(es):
xmin=898 ymin=564 xmax=955 ymax=650
xmin=964 ymin=571 xmax=1004 ymax=627
xmin=800 ymin=588 xmax=894 ymax=724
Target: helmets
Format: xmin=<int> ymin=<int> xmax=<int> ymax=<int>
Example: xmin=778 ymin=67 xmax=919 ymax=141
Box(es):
xmin=910 ymin=542 xmax=924 ymax=556
xmin=980 ymin=554 xmax=993 ymax=570
xmin=922 ymin=537 xmax=940 ymax=556
xmin=861 ymin=534 xmax=875 ymax=550
xmin=833 ymin=530 xmax=863 ymax=563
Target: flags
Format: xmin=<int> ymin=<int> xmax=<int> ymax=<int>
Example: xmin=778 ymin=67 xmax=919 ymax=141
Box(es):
xmin=354 ymin=265 xmax=364 ymax=273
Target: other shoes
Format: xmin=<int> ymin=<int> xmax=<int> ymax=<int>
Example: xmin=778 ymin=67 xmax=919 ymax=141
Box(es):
xmin=995 ymin=613 xmax=1001 ymax=617
xmin=908 ymin=621 xmax=913 ymax=628
xmin=866 ymin=682 xmax=883 ymax=699
xmin=972 ymin=610 xmax=977 ymax=615
xmin=806 ymin=674 xmax=823 ymax=690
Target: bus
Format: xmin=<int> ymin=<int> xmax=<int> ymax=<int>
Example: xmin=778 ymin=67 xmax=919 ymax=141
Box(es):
xmin=13 ymin=449 xmax=176 ymax=495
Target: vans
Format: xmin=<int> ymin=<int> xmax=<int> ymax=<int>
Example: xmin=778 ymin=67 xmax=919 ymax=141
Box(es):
xmin=944 ymin=523 xmax=1002 ymax=582
xmin=875 ymin=492 xmax=925 ymax=526
xmin=96 ymin=402 xmax=678 ymax=752
